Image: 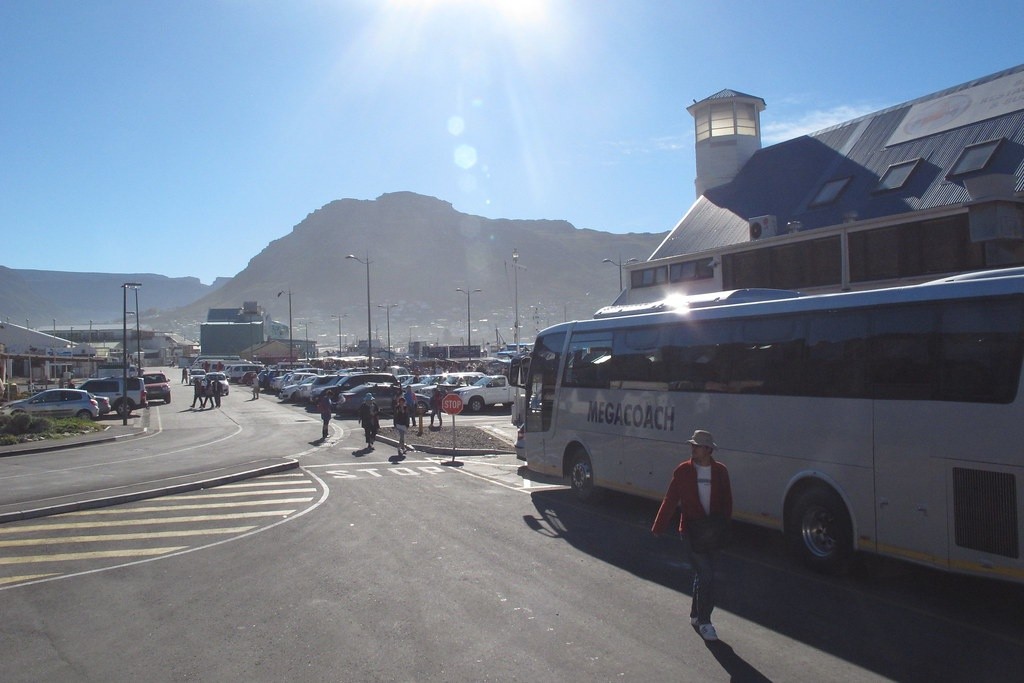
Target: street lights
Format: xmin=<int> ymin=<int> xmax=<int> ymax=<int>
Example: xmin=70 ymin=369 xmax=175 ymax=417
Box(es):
xmin=276 ymin=290 xmax=294 ymax=369
xmin=455 ymin=286 xmax=485 ymax=372
xmin=71 ymin=326 xmax=74 ymax=358
xmin=243 ymin=311 xmax=257 ymax=364
xmin=300 ymin=322 xmax=314 ymax=363
xmin=87 ymin=320 xmax=94 ymax=379
xmin=510 ymin=247 xmax=526 ymax=357
xmin=347 ymin=252 xmax=376 ymax=373
xmin=26 ymin=318 xmax=34 ymax=397
xmin=53 ymin=318 xmax=56 ymax=386
xmin=602 ymin=252 xmax=639 ymax=295
xmin=329 ymin=313 xmax=349 ymax=360
xmin=125 ymin=282 xmax=143 ymax=376
xmin=377 ymin=303 xmax=398 ymax=363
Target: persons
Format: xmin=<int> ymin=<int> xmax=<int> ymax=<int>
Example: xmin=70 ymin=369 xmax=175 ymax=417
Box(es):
xmin=190 ymin=378 xmax=222 ymax=409
xmin=320 ymin=390 xmax=334 ymax=438
xmin=413 ymin=374 xmax=419 ymax=384
xmin=252 ymin=373 xmax=260 ymax=400
xmin=67 ymin=378 xmax=75 ymax=389
xmin=392 ymin=385 xmax=443 ymax=428
xmin=181 ymin=366 xmax=188 ymax=383
xmin=216 ymin=361 xmax=224 ymax=371
xmin=359 ymin=393 xmax=380 ymax=450
xmin=203 ymin=360 xmax=209 ymax=373
xmin=394 ymin=398 xmax=410 ymax=456
xmin=651 ymin=431 xmax=734 ymax=640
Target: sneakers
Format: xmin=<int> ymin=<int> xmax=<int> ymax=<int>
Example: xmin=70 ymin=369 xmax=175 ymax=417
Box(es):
xmin=699 ymin=624 xmax=718 ymax=641
xmin=690 ymin=617 xmax=714 ymax=628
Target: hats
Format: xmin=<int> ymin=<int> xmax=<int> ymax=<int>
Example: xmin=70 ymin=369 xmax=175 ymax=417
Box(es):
xmin=363 ymin=393 xmax=375 ymax=401
xmin=684 ymin=430 xmax=717 ymax=449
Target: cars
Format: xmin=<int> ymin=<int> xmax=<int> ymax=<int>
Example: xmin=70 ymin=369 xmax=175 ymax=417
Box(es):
xmin=88 ymin=393 xmax=112 ymax=418
xmin=189 ymin=354 xmax=486 ymax=413
xmin=336 ymin=381 xmax=434 ymax=419
xmin=188 ymin=369 xmax=207 ymax=384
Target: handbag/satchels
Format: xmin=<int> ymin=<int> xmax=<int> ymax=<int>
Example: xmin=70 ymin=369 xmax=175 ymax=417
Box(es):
xmin=396 ymin=424 xmax=407 ymax=432
xmin=685 ymin=518 xmax=733 ymax=553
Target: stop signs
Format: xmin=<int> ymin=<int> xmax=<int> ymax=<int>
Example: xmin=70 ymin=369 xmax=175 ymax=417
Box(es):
xmin=443 ymin=394 xmax=463 ymax=415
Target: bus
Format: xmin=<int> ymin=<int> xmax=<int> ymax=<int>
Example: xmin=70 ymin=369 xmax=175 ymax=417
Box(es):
xmin=511 ymin=265 xmax=1024 ymax=594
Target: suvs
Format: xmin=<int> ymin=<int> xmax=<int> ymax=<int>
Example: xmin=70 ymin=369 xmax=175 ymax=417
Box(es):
xmin=142 ymin=370 xmax=173 ymax=403
xmin=204 ymin=372 xmax=230 ymax=395
xmin=1 ymin=388 xmax=100 ymax=424
xmin=74 ymin=376 xmax=149 ymax=420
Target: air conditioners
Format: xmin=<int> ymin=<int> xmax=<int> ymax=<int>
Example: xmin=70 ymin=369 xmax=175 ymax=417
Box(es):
xmin=748 ymin=214 xmax=779 ymax=241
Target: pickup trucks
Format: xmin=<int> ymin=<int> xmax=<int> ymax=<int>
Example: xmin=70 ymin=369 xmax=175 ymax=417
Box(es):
xmin=453 ymin=375 xmax=526 ymax=412
